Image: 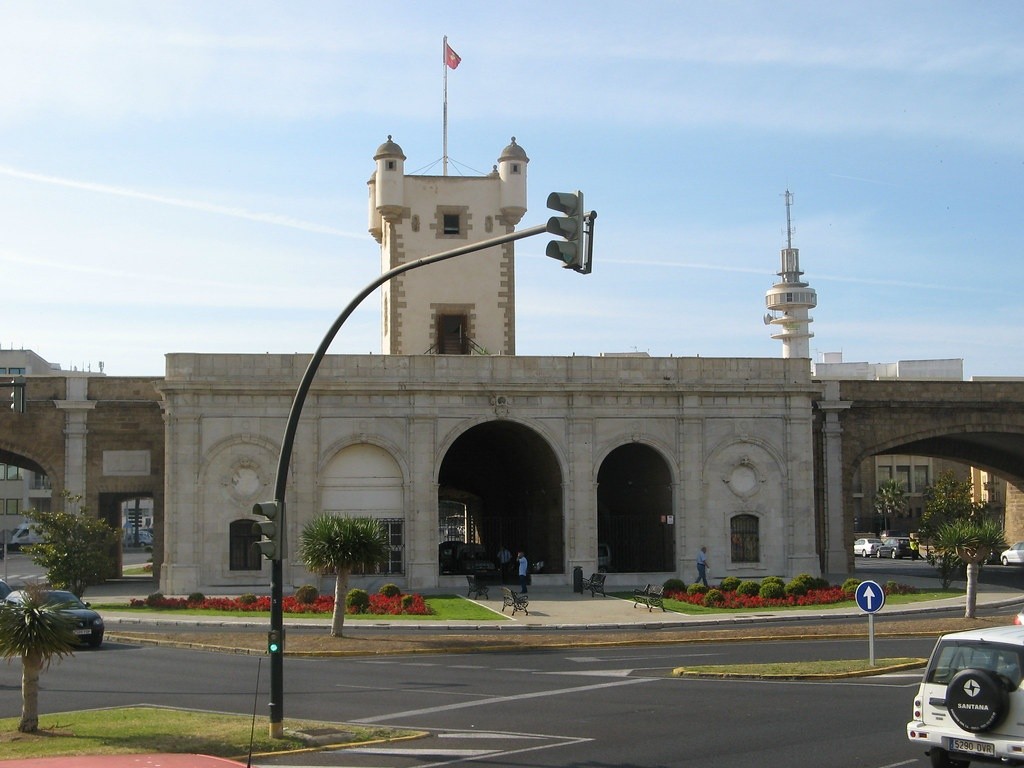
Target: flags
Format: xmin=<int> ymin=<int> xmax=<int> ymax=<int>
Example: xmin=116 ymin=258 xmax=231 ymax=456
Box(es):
xmin=447 ymin=44 xmax=461 ymax=70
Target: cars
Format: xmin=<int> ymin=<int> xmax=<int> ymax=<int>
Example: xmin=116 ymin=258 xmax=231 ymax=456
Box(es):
xmin=0 ymin=578 xmax=105 ymax=649
xmin=853 ymin=537 xmax=884 ymax=558
xmin=1000 ymin=541 xmax=1024 ymax=566
xmin=133 ymin=530 xmax=154 ymax=548
xmin=439 ymin=540 xmax=506 ymax=583
xmin=875 ymin=536 xmax=912 ymax=560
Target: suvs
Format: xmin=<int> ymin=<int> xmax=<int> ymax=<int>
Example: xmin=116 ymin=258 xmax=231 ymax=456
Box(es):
xmin=906 ymin=624 xmax=1024 ymax=768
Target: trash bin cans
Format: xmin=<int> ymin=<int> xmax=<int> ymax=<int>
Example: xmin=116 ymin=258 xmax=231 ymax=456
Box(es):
xmin=573 ymin=566 xmax=583 ymax=592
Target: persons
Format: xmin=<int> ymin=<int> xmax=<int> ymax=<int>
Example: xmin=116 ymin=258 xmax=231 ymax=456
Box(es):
xmin=498 ymin=547 xmax=512 ymax=568
xmin=910 ymin=539 xmax=919 ymax=561
xmin=695 ymin=547 xmax=710 ymax=585
xmin=517 ymin=552 xmax=528 ymax=593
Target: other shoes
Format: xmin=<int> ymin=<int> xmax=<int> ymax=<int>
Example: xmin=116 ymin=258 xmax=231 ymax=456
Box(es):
xmin=520 ymin=590 xmax=527 ymax=594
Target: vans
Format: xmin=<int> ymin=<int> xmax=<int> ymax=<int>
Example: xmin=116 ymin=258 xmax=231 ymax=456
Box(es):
xmin=531 ymin=539 xmax=612 ymax=573
xmin=5 ymin=521 xmax=56 ymax=552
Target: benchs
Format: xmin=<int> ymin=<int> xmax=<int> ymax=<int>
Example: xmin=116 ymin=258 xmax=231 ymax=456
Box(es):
xmin=581 ymin=572 xmax=606 ymax=598
xmin=500 ymin=586 xmax=529 ymax=616
xmin=464 ymin=575 xmax=488 ymax=600
xmin=633 ymin=585 xmax=666 ymax=613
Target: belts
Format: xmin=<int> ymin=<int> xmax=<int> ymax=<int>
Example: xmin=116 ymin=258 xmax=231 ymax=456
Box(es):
xmin=697 ymin=563 xmax=705 ymax=566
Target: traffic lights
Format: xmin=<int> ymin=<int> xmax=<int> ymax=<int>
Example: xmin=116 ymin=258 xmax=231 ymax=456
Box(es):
xmin=545 ymin=189 xmax=584 ymax=269
xmin=252 ymin=500 xmax=282 ymax=561
